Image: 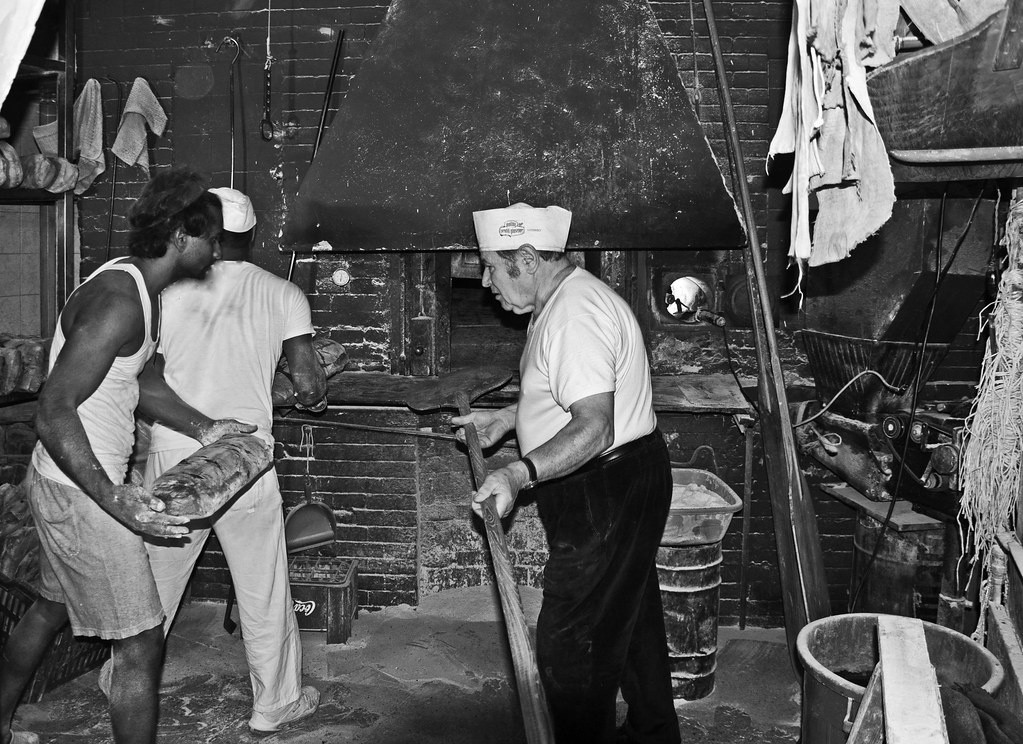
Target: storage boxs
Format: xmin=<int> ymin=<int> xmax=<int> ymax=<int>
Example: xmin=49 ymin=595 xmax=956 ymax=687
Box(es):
xmin=239 ymin=557 xmax=361 ymax=643
xmin=0 ymin=573 xmax=112 ymax=704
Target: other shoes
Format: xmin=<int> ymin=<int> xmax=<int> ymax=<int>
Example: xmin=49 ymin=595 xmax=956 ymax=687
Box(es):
xmin=10 ymin=729 xmax=40 ymax=744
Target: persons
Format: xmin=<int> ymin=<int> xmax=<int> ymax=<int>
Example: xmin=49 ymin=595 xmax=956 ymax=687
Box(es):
xmin=452 ymin=203 xmax=681 ymax=744
xmin=98 ymin=187 xmax=325 ymax=736
xmin=1 ymin=166 xmax=256 ymax=744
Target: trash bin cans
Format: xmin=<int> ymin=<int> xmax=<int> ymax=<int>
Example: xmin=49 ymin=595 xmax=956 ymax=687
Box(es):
xmin=795 ymin=613 xmax=1006 ymax=744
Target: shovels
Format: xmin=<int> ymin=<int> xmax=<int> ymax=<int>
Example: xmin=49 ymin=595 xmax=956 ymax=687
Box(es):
xmin=406 ymin=366 xmax=555 ymax=744
xmin=284 ymin=472 xmax=337 ymax=555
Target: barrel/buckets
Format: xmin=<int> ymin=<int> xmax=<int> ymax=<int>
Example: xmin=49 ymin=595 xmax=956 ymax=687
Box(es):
xmin=797 ymin=613 xmax=1004 ymax=744
xmin=655 ymin=540 xmax=723 ymax=701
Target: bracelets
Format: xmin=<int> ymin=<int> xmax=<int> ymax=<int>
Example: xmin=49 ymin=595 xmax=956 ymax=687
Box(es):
xmin=522 ymin=457 xmax=538 ymax=489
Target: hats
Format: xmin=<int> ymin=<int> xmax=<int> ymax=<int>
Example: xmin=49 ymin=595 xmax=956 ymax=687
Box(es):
xmin=472 ymin=202 xmax=573 ymax=252
xmin=207 ymin=187 xmax=257 ymax=233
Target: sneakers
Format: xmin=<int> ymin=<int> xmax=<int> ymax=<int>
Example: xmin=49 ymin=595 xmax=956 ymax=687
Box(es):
xmin=247 ymin=686 xmax=320 ymax=733
xmin=98 ymin=657 xmax=115 ymax=699
